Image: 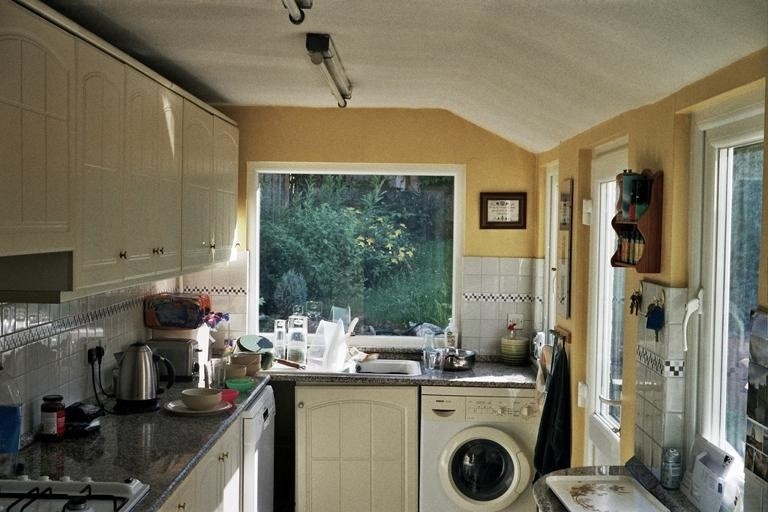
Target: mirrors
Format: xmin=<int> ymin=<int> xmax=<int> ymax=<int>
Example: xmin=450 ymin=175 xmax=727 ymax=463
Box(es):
xmin=555 ymin=178 xmax=573 ymax=320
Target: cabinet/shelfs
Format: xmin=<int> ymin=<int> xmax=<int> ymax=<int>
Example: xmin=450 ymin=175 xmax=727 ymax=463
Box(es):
xmin=611 ymin=170 xmax=663 ymax=273
xmin=195 ymin=416 xmax=240 ymax=512
xmin=1 ymin=29 xmax=183 ymax=305
xmin=294 ymin=376 xmax=418 ymax=512
xmin=157 ymin=468 xmax=197 ymax=512
xmin=183 ymin=91 xmax=239 ymax=275
xmin=0 ymin=1 xmax=78 ymax=256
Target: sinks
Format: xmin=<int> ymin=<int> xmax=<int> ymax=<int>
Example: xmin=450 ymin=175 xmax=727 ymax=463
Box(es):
xmin=349 ymin=358 xmax=422 ymax=377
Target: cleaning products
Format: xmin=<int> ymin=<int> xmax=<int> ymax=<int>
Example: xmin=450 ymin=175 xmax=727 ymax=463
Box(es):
xmin=444 ymin=318 xmax=457 ymax=350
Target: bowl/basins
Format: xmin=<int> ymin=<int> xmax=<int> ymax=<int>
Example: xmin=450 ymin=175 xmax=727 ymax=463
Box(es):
xmin=430 ymin=348 xmax=476 ymax=371
xmin=225 ymin=378 xmax=253 ymax=393
xmin=231 ymin=365 xmax=247 ymax=378
xmin=181 ymin=388 xmax=222 ymax=410
xmin=221 ymin=389 xmax=239 ymax=401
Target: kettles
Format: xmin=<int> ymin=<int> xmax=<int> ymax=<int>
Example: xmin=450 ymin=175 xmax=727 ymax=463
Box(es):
xmin=87 ymin=342 xmax=175 ymax=415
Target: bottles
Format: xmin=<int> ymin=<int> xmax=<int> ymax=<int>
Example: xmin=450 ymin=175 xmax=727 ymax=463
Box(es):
xmin=287 ymin=306 xmax=308 ymax=367
xmin=39 ymin=395 xmax=66 ymax=440
xmin=0 ymin=363 xmax=24 ymax=478
xmin=445 ymin=318 xmax=459 ymax=349
xmin=272 ymin=319 xmax=287 ymax=362
xmin=434 ymin=331 xmax=445 ymax=348
xmin=421 ymin=332 xmax=434 ymax=353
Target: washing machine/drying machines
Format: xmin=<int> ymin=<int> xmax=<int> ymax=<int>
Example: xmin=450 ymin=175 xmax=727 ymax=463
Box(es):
xmin=417 ymin=384 xmax=545 ymax=512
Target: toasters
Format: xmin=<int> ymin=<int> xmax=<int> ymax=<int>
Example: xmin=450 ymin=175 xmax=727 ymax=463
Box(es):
xmin=146 ymin=338 xmax=202 ymax=382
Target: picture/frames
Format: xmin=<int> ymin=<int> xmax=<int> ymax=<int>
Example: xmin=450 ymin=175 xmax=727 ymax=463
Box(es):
xmin=480 ymin=192 xmax=527 ymax=230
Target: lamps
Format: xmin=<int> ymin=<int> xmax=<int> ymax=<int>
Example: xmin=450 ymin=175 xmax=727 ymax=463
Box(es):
xmin=305 ymin=33 xmax=353 ymax=109
xmin=280 ymin=0 xmax=314 ymax=25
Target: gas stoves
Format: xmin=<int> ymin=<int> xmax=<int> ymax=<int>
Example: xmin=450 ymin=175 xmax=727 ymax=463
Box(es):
xmin=0 ymin=474 xmax=150 ymax=512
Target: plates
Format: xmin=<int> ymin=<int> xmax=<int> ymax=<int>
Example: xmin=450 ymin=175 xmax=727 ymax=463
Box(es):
xmin=499 ymin=337 xmax=530 ymax=366
xmin=163 ymin=399 xmax=232 ymax=416
xmin=545 ymin=475 xmax=670 ymax=512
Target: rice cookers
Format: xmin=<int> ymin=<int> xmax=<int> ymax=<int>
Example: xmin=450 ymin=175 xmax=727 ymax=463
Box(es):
xmin=231 ymin=352 xmax=261 ymax=377
